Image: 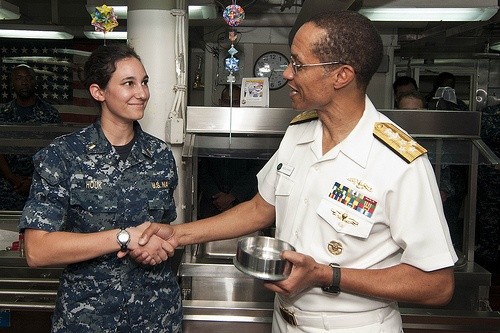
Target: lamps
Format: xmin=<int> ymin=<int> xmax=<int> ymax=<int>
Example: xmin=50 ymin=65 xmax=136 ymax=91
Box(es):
xmin=84 ymin=25 xmax=128 ymax=40
xmin=0 ymin=0 xmax=20 ymax=21
xmin=357 ymin=0 xmax=499 ymax=22
xmin=187 ymin=0 xmax=217 ymax=19
xmin=0 ymin=24 xmax=74 ymax=41
xmin=85 ymin=0 xmax=129 ymax=19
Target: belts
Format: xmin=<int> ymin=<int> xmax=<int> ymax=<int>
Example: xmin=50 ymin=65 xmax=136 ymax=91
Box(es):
xmin=275 ymin=299 xmax=394 ymax=327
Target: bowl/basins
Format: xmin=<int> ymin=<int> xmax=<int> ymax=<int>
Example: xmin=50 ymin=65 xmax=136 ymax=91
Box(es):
xmin=233 ymin=236 xmax=296 ymax=280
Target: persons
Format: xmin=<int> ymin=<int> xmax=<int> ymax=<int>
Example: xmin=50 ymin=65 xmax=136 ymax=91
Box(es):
xmin=17 ymin=45 xmax=185 ymax=332
xmin=392 ymin=71 xmax=500 ymax=202
xmin=200 ymin=85 xmax=268 ymax=220
xmin=1 ymin=63 xmax=61 ymax=212
xmin=130 ymin=10 xmax=458 ymax=333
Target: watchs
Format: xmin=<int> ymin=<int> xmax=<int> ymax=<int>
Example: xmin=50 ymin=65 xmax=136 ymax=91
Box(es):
xmin=322 ymin=261 xmax=342 ymax=295
xmin=115 ymin=225 xmax=131 ymax=251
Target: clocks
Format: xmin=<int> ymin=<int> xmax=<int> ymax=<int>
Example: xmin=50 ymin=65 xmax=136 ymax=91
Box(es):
xmin=254 ymin=51 xmax=290 ymax=90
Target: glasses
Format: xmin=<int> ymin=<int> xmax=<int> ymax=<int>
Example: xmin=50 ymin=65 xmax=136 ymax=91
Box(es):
xmin=289 ymin=57 xmax=340 ymax=74
